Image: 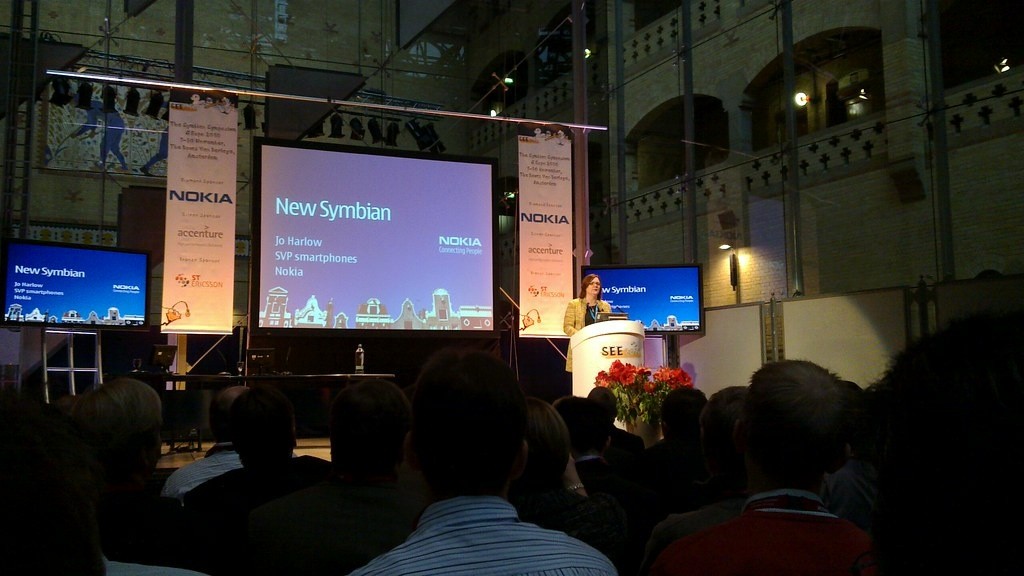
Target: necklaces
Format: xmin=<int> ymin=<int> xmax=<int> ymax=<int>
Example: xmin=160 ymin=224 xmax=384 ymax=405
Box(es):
xmin=591 ymin=306 xmax=595 ymax=312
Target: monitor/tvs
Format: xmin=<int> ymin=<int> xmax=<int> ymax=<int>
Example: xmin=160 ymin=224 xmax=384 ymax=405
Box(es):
xmin=582 ymin=264 xmax=706 ymax=335
xmin=0 ymin=238 xmax=152 ymax=332
xmin=248 ymin=347 xmax=275 ymax=369
xmin=149 ymin=344 xmax=178 ymax=372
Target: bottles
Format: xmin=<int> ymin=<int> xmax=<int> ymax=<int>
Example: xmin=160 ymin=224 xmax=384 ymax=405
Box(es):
xmin=356 ymin=344 xmax=364 ymax=374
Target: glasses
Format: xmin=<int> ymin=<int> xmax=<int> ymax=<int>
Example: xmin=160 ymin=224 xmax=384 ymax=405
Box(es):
xmin=589 ymin=282 xmax=601 ymax=285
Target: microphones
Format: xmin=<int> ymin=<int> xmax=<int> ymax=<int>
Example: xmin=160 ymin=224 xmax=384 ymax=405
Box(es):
xmin=617 ymin=306 xmax=624 ymax=313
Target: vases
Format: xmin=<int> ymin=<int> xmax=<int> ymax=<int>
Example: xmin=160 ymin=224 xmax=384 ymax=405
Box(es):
xmin=626 ymin=417 xmax=662 ymax=448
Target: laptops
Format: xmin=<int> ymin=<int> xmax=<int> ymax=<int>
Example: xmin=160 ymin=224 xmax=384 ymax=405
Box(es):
xmin=596 ymin=312 xmax=628 ymax=323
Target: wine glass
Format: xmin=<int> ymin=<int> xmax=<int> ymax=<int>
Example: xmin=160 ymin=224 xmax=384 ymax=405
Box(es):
xmin=237 ymin=362 xmax=244 ymax=376
xmin=132 ymin=359 xmax=141 ymax=372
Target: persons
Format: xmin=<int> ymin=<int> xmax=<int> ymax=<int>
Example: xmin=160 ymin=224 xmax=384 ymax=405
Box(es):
xmin=650 ymin=361 xmax=877 ymax=576
xmin=563 ymin=274 xmax=613 ymax=373
xmin=345 ymin=350 xmax=618 ymax=576
xmin=0 ymin=377 xmax=881 ymax=576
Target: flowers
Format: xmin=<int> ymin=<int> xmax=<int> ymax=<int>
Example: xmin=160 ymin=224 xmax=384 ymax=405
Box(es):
xmin=593 ymin=359 xmax=694 ymax=433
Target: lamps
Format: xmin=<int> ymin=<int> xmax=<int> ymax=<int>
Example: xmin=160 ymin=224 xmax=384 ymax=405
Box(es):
xmin=404 ymin=119 xmax=432 ymax=150
xmin=243 ymin=105 xmax=260 ymax=131
xmin=384 ymin=122 xmax=401 ymax=147
xmin=74 ymin=83 xmax=96 ymax=111
xmin=47 ymin=79 xmax=73 ymax=109
xmin=142 ymin=92 xmax=164 ymax=121
xmin=307 ymin=121 xmax=325 ymax=139
xmin=367 ymin=119 xmax=384 ymax=144
xmin=123 ymin=88 xmax=141 ymax=117
xmin=328 ymin=114 xmax=345 ymax=138
xmin=349 ymin=117 xmax=366 ymax=140
xmin=421 ymin=123 xmax=446 ymax=153
xmin=99 ymin=87 xmax=119 ymax=114
xmin=717 ymin=232 xmax=733 ymax=250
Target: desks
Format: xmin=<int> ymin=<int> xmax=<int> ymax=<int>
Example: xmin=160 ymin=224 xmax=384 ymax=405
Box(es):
xmin=129 ymin=369 xmax=396 ymax=455
